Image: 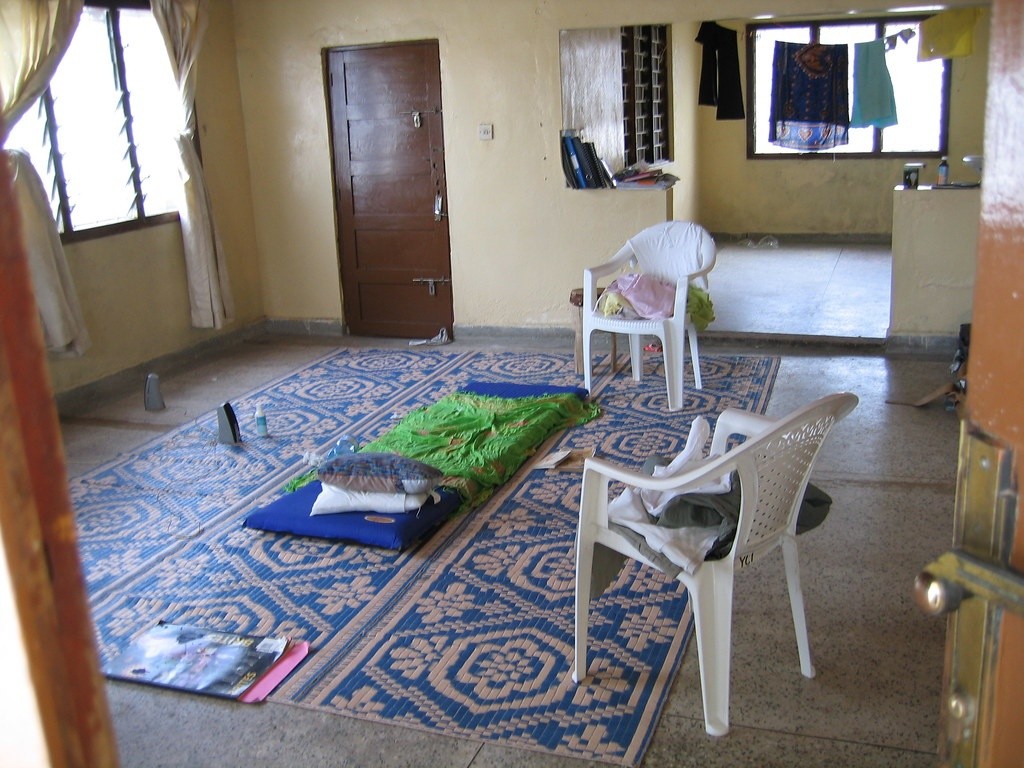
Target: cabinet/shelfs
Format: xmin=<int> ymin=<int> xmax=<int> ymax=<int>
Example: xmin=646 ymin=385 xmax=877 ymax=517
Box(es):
xmin=621 ymin=25 xmax=670 ymax=168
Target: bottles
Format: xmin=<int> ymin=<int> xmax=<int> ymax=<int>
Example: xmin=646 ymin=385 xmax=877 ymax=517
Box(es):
xmin=937 ymin=157 xmax=948 ymax=184
xmin=255 ymin=405 xmax=268 ymax=437
xmin=328 ymin=433 xmax=360 ymax=460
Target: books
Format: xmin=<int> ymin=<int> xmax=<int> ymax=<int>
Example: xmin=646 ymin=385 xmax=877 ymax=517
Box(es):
xmin=560 ymin=128 xmax=662 ymax=189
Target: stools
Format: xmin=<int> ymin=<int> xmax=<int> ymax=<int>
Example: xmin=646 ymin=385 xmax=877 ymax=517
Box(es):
xmin=570 ymin=287 xmax=618 ymax=375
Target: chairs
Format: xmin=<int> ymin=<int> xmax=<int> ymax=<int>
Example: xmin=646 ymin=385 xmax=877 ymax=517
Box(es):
xmin=584 ymin=219 xmax=718 ymax=412
xmin=575 ymin=392 xmax=861 ymax=736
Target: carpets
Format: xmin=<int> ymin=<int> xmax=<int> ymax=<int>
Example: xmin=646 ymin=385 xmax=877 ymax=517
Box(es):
xmin=72 ymin=347 xmax=783 ymax=768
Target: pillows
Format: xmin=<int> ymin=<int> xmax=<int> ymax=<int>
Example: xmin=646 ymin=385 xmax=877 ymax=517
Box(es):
xmin=317 ymin=452 xmax=444 ymax=495
xmin=310 ymin=481 xmax=432 ymax=517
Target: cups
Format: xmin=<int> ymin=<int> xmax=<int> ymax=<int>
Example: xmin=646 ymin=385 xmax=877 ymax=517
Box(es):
xmin=902 ymin=167 xmax=919 ymax=190
